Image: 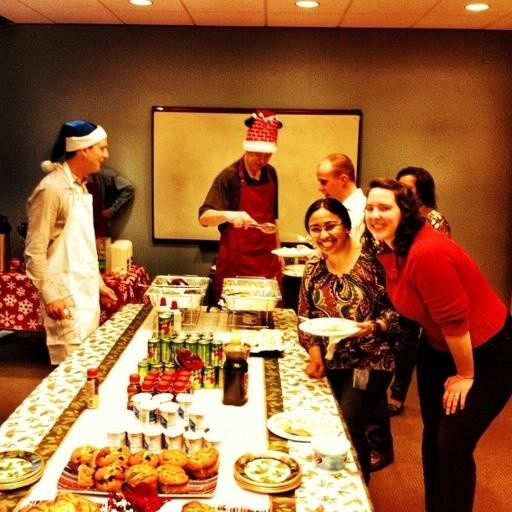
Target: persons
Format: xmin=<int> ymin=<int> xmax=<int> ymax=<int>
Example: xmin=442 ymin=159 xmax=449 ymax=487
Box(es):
xmin=24 ymin=120 xmax=118 ymax=368
xmin=358 ymin=175 xmax=512 ymax=512
xmin=306 ymin=152 xmax=369 ymax=246
xmin=86 ymin=164 xmax=136 ymax=243
xmin=295 ymin=197 xmax=394 ymax=488
xmin=198 ymin=109 xmax=285 ymax=308
xmin=386 ymin=166 xmax=454 ymax=418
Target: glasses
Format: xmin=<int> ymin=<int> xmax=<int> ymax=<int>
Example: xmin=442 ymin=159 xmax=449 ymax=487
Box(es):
xmin=307 ymin=221 xmax=343 ymax=233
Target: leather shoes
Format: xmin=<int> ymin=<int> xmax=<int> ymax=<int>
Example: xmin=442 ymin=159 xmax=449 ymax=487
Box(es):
xmin=388 ymin=402 xmax=403 ymax=418
xmin=371 ymin=449 xmax=394 ymax=472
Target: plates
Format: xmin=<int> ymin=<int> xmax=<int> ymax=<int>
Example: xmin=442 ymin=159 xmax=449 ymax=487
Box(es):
xmin=297 ymin=317 xmax=363 ymax=337
xmin=0 ymin=449 xmax=46 ymax=491
xmin=56 ymin=489 xmax=216 ymax=498
xmin=264 ymin=410 xmax=341 ymax=443
xmin=233 ymin=449 xmax=304 ymax=494
xmin=281 ymin=270 xmax=304 ymax=278
xmin=270 ymin=247 xmax=317 ymax=258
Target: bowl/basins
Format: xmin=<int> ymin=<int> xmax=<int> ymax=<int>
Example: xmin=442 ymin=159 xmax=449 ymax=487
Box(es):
xmin=144 ymin=286 xmax=206 ymax=308
xmin=150 ymin=276 xmax=211 ymax=288
xmin=313 ymin=439 xmax=352 ymax=471
xmin=286 ymin=264 xmax=304 ymax=274
xmin=221 ymin=278 xmax=283 ymax=313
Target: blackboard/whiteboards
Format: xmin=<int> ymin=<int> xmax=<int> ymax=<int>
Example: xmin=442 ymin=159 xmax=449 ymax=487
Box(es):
xmin=151 ymin=105 xmax=363 ymax=247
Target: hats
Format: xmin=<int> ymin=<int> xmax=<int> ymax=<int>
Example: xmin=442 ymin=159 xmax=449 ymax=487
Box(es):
xmin=243 ymin=108 xmax=284 ymax=154
xmin=40 ymin=119 xmax=108 ymax=174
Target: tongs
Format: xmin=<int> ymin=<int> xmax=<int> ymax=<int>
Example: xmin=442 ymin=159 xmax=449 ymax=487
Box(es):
xmin=248 ymin=222 xmax=279 ymax=234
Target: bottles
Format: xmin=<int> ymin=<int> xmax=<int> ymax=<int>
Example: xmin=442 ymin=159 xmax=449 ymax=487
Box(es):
xmin=172 ymin=331 xmax=214 ymax=342
xmin=160 ymin=338 xmax=171 ymax=363
xmin=152 ymin=364 xmax=163 ymax=372
xmin=128 ymin=373 xmax=141 ymax=411
xmin=147 ymin=338 xmax=160 ymax=364
xmin=191 ymin=369 xmax=202 ymax=389
xmin=211 ymin=340 xmax=225 ymax=366
xmin=172 ymin=338 xmax=184 ymax=363
xmin=222 ymin=346 xmax=249 ymax=406
xmin=138 ymin=361 xmax=150 ymax=390
xmin=158 ymin=297 xmax=169 ymax=314
xmin=141 ymin=370 xmax=193 ymax=402
xmin=86 ymin=369 xmax=99 ymax=409
xmin=170 ymin=301 xmax=182 ymax=334
xmin=164 ymin=363 xmax=175 ymax=373
xmin=158 ymin=313 xmax=171 ymax=338
xmin=186 ymin=338 xmax=197 ymax=354
xmin=198 ymin=340 xmax=211 ymax=365
xmin=203 ymin=366 xmax=215 ymax=389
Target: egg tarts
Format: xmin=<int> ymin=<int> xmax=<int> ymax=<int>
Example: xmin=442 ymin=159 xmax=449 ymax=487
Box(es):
xmin=158 ymin=451 xmax=187 ymax=468
xmin=186 ymin=448 xmax=219 ymax=479
xmin=72 ymin=446 xmax=99 ymax=471
xmin=123 ymin=465 xmax=158 ymax=495
xmin=77 ymin=465 xmax=95 ymax=489
xmin=156 ymin=465 xmax=189 ymax=495
xmin=96 ymin=448 xmax=131 ymax=470
xmin=95 ymin=465 xmax=126 ymax=492
xmin=128 ymin=451 xmax=159 ymax=468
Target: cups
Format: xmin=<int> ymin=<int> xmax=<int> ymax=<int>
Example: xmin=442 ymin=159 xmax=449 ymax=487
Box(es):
xmin=112 ymin=244 xmax=129 ymax=278
